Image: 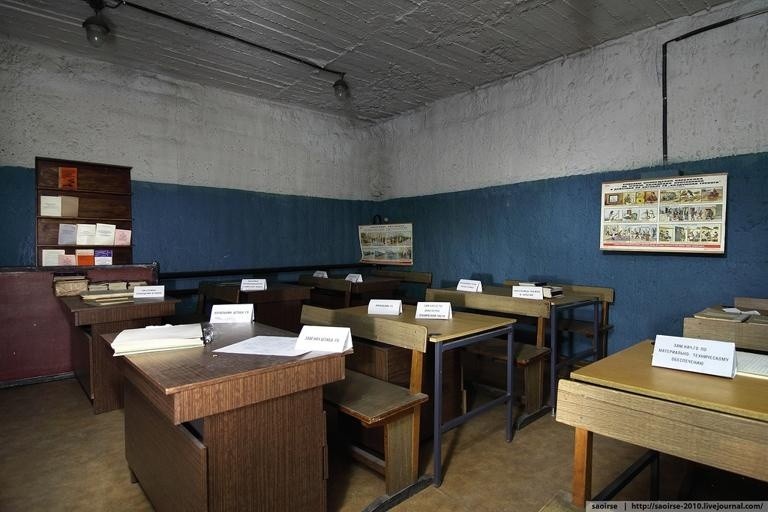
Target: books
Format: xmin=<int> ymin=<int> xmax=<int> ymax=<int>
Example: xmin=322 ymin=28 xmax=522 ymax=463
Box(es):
xmin=61 ymin=196 xmax=80 ymax=217
xmin=52 ymin=274 xmax=147 ymax=306
xmin=58 ymin=167 xmax=77 ymax=190
xmin=41 ymin=222 xmax=132 ymax=267
xmin=693 ymin=305 xmax=750 ymax=322
xmin=110 ymin=322 xmax=206 ymax=357
xmin=40 ymin=195 xmax=61 ymax=216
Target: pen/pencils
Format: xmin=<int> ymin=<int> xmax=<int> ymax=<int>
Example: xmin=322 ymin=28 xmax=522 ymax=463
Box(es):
xmin=720 ymin=305 xmax=735 ymax=308
xmin=651 ymin=342 xmax=655 ymax=345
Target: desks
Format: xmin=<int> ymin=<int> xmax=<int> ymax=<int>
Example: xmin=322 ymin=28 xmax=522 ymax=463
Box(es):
xmin=57 ymin=270 xmax=768 ymax=512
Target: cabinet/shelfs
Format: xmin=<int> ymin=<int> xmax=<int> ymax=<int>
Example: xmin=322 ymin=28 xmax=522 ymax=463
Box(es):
xmin=34 ymin=154 xmax=133 ymax=266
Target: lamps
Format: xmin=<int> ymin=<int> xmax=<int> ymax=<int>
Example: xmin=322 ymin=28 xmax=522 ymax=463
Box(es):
xmin=333 ymin=72 xmax=351 ymax=101
xmin=80 ymin=0 xmax=111 ymax=48
